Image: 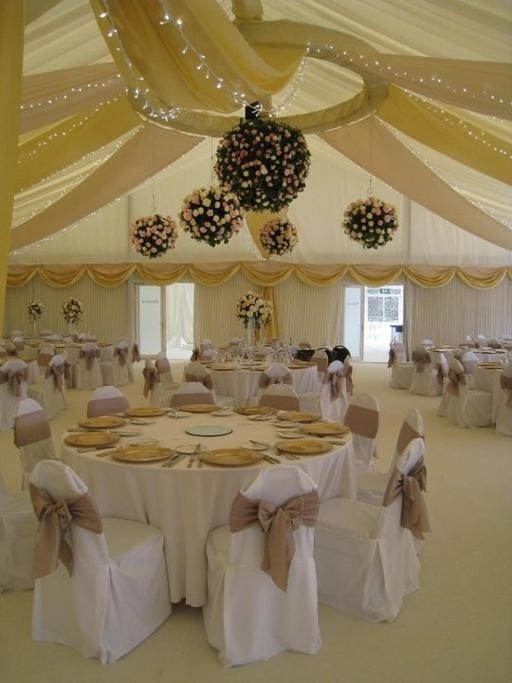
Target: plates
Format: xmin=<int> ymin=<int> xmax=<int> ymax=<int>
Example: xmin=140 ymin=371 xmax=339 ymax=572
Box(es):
xmin=64 ymin=401 xmax=349 ymax=440
xmin=113 ymin=446 xmax=174 ymax=462
xmin=128 ymin=437 xmax=160 ymax=446
xmin=203 ymin=340 xmax=315 ymax=371
xmin=199 ymin=448 xmax=265 ymax=465
xmin=65 ymin=431 xmax=122 ymax=447
xmin=479 ymin=360 xmax=503 ymax=370
xmin=176 ymin=444 xmax=208 ymax=454
xmin=273 ymin=439 xmax=333 ymax=454
xmin=241 ymin=440 xmax=272 ymax=450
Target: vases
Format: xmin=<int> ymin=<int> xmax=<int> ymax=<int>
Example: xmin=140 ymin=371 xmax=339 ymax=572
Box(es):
xmin=67 ymin=318 xmax=75 ymax=335
xmin=33 ymin=318 xmax=38 ymax=338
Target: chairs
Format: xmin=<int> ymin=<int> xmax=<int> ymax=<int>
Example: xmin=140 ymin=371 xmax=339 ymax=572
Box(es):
xmin=259 ymin=382 xmax=301 ymax=412
xmin=354 ymin=407 xmax=426 ymax=507
xmin=1 ymin=329 xmax=511 ymax=408
xmin=1 ymin=358 xmax=29 ymax=431
xmin=495 ymin=366 xmax=512 ymax=436
xmin=28 ymin=354 xmax=67 ymax=422
xmin=432 ymin=353 xmax=492 ymax=431
xmin=299 ymin=360 xmax=348 ymax=423
xmin=85 ymin=384 xmax=130 ymax=417
xmin=27 ymin=459 xmax=173 ymax=666
xmin=201 ymin=464 xmax=325 ymax=670
xmin=341 ymin=391 xmax=381 ymax=472
xmin=0 ymin=488 xmax=39 ymax=593
xmin=10 ymin=397 xmax=58 ymax=491
xmin=314 ymin=435 xmax=431 ymax=626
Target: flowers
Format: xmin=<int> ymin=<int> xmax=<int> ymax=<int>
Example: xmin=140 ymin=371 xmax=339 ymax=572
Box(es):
xmin=256 ymin=215 xmax=301 ymax=256
xmin=21 ymin=296 xmax=49 ymax=320
xmin=56 ymin=293 xmax=86 ymax=324
xmin=234 ymin=289 xmax=273 ymax=331
xmin=338 ymin=193 xmax=401 ymax=251
xmin=125 ymin=211 xmax=180 ymax=257
xmin=176 ymin=178 xmax=244 ymax=246
xmin=212 ymin=115 xmax=312 ymax=214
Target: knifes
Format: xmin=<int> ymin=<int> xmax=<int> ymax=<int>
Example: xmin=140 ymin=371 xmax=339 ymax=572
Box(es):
xmin=77 ymin=445 xmax=115 ymax=453
xmin=162 ymin=452 xmax=186 ymax=468
xmin=186 ymin=454 xmax=195 ymax=468
